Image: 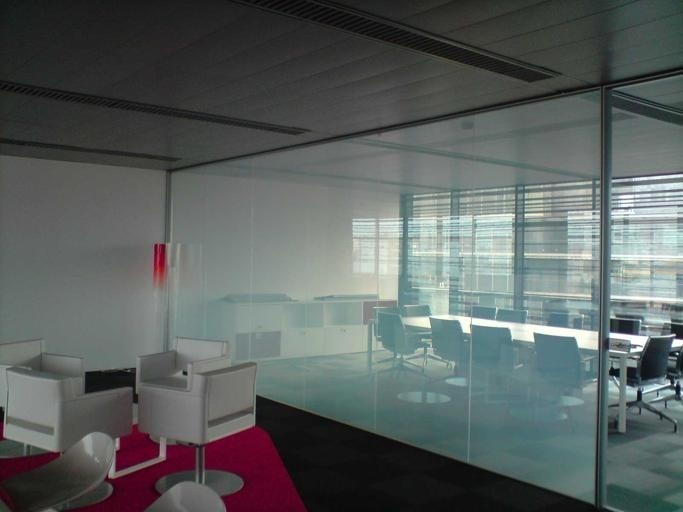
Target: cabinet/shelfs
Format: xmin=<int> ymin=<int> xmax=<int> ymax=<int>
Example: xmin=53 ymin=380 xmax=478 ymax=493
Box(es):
xmin=204 ymin=294 xmax=397 ymax=366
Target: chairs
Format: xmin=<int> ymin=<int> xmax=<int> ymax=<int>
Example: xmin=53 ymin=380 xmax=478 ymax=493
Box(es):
xmin=368 ymin=305 xmax=682 ymax=431
xmin=3 ymin=338 xmax=257 ymax=496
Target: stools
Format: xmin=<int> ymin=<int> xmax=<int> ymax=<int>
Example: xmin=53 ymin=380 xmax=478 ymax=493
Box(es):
xmin=143 ymin=480 xmax=227 ymax=512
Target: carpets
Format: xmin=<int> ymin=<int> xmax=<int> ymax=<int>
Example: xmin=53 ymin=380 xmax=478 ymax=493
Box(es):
xmin=1 ymin=427 xmax=309 ymax=510
